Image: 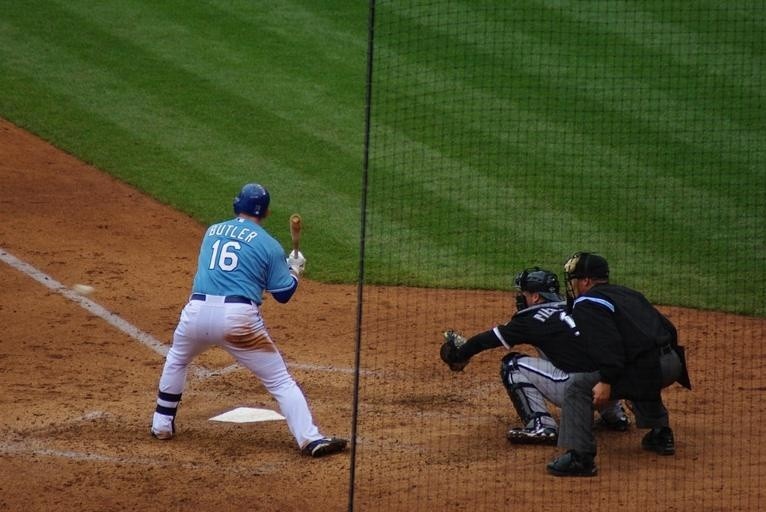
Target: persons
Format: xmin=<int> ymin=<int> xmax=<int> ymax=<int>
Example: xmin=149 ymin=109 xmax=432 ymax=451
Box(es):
xmin=545 ymin=251 xmax=691 ymax=476
xmin=439 ymin=266 xmax=633 ymax=444
xmin=151 ymin=183 xmax=347 ymax=457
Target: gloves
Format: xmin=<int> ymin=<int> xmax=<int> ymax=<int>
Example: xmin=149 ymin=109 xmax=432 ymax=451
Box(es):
xmin=284 ymin=248 xmax=307 ymax=278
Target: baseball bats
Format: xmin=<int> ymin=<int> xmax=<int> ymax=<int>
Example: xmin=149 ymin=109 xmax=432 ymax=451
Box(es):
xmin=289 ymin=214 xmax=302 ymax=259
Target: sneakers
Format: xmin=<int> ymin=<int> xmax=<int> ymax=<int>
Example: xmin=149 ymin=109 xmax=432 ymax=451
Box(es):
xmin=600 ymin=404 xmax=631 ymax=431
xmin=506 ymin=420 xmax=561 ymax=447
xmin=548 ymin=453 xmax=603 ymax=480
xmin=304 ymin=433 xmax=349 ymax=459
xmin=148 ymin=424 xmax=177 ymax=440
xmin=641 ymin=427 xmax=679 ymax=457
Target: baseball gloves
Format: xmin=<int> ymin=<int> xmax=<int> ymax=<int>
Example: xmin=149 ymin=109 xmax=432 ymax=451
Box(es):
xmin=439 ymin=328 xmax=469 ymax=372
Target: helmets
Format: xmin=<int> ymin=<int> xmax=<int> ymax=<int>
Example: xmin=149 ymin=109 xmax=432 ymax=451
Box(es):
xmin=231 ymin=182 xmax=271 ymax=219
xmin=514 ymin=265 xmax=564 ymax=303
xmin=564 ymin=252 xmax=609 ymax=290
